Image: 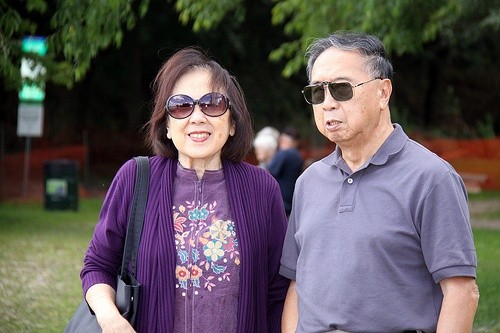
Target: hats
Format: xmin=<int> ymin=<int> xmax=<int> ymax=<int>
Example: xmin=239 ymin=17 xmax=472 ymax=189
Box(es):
xmin=253 ymin=125 xmax=280 ymax=148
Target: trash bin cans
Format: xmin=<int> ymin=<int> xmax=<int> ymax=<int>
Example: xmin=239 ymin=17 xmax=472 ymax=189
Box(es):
xmin=42 ymin=160 xmax=80 ymax=212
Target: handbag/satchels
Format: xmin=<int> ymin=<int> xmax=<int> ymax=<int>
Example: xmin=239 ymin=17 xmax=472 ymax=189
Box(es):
xmin=64 ymin=156 xmax=150 ymax=333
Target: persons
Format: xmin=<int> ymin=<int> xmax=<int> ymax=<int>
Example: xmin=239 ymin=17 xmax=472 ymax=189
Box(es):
xmin=79 ymin=48 xmax=298 ymax=333
xmin=278 ymin=29 xmax=480 ymax=333
xmin=253 ymin=126 xmax=305 ymax=216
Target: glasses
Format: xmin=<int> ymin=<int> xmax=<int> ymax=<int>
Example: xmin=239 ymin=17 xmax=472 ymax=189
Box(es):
xmin=300 ymin=76 xmax=387 ymax=104
xmin=162 ymin=92 xmax=235 ymax=120
